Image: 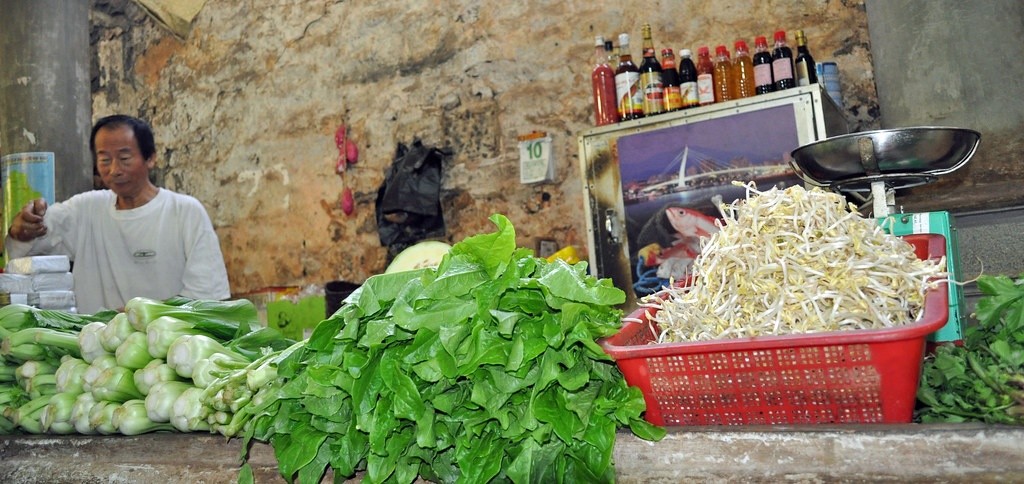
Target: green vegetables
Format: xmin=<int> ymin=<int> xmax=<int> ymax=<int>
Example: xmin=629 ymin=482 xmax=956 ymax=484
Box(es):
xmin=0 ymin=213 xmax=669 ymax=484
xmin=913 ymin=270 xmax=1024 ymax=426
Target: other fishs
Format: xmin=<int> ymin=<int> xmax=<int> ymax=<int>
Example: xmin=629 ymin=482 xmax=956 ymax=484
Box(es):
xmin=656 ymin=206 xmax=723 ymax=286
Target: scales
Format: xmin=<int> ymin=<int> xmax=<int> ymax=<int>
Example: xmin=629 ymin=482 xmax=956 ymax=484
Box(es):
xmin=788 ymin=126 xmax=983 ymax=342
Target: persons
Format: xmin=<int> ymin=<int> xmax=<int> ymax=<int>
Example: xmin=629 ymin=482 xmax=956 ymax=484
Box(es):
xmin=3 ymin=115 xmax=230 ymax=316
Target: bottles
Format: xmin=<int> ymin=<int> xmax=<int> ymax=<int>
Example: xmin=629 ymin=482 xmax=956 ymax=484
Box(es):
xmin=679 ymin=30 xmax=815 ymax=109
xmin=604 ymin=41 xmax=618 ymax=72
xmin=615 ymin=33 xmax=643 ymax=123
xmin=661 ymin=49 xmax=680 ymax=113
xmin=592 ymin=35 xmax=618 ymax=126
xmin=639 ymin=24 xmax=664 ymax=117
